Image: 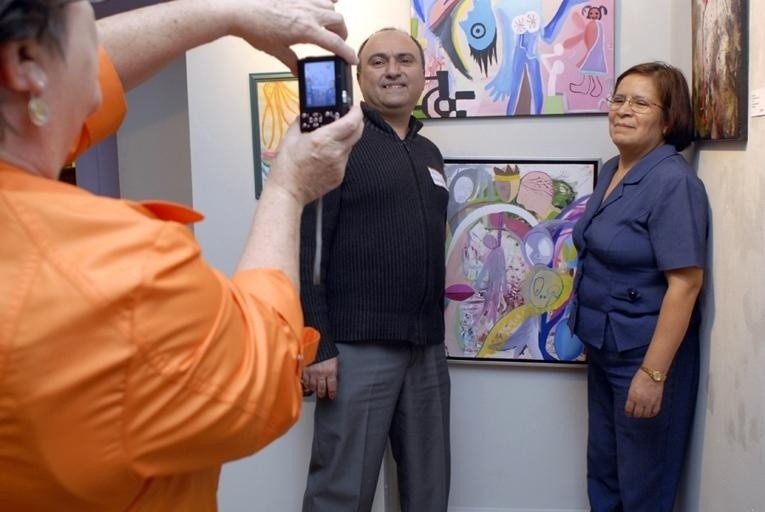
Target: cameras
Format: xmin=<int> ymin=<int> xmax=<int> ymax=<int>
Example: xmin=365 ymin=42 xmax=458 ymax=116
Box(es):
xmin=298 ymin=54 xmax=353 ymax=133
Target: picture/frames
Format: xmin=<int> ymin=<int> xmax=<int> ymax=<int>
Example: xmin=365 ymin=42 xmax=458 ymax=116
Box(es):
xmin=436 ymin=158 xmax=601 ymax=368
xmin=249 ymin=69 xmax=300 ymax=205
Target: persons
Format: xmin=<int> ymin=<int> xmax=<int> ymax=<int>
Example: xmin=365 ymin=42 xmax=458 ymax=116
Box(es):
xmin=325 ymin=79 xmax=336 ymax=106
xmin=0 ymin=0 xmax=368 ymax=512
xmin=305 ymin=77 xmax=315 ymax=108
xmin=567 ymin=57 xmax=712 ymax=512
xmin=296 ymin=26 xmax=454 ymax=512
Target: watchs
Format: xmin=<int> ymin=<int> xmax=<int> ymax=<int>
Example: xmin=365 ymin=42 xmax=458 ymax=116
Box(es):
xmin=638 ymin=364 xmax=669 ymax=383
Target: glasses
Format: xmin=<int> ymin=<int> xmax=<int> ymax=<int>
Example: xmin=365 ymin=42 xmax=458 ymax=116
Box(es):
xmin=606 ymin=96 xmax=666 ymax=114
